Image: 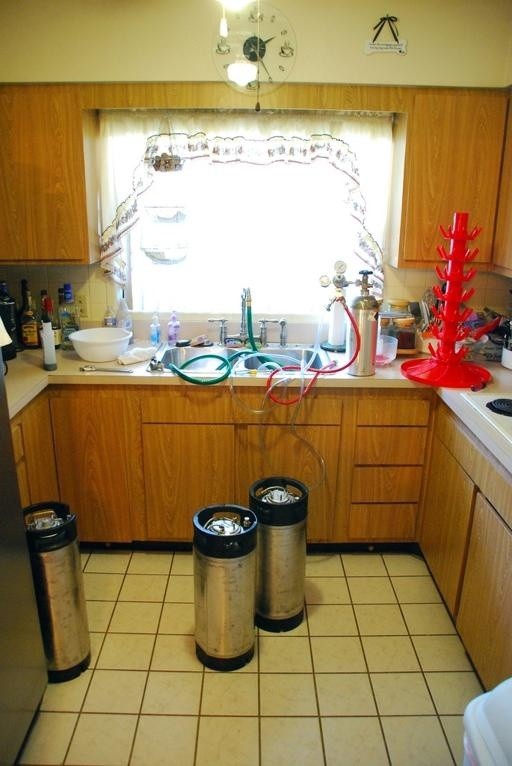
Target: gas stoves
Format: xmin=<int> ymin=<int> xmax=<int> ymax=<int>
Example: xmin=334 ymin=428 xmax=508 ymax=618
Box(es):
xmin=462 ymin=390 xmax=512 ymax=445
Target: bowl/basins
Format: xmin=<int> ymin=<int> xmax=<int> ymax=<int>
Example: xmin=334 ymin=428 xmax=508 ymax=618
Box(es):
xmin=376 ymin=334 xmax=398 ymax=365
xmin=67 ymin=327 xmax=133 ymax=362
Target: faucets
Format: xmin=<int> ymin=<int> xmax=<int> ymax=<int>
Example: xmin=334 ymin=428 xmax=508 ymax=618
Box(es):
xmin=279 ymin=318 xmax=289 ymax=347
xmin=241 ymin=288 xmax=252 ymax=334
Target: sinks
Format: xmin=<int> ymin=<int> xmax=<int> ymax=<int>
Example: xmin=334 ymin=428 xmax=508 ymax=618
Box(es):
xmin=243 ymin=344 xmax=334 ymax=376
xmin=146 ymin=341 xmax=241 ymax=376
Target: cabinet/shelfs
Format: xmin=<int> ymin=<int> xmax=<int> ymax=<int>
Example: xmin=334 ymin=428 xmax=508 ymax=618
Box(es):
xmin=0 ymin=89 xmax=104 ymax=265
xmin=384 ymin=84 xmax=502 ymax=275
xmin=419 ymin=408 xmax=511 ymax=695
xmin=139 ymin=389 xmax=341 ymax=550
xmin=351 ymin=396 xmax=426 ymax=542
xmin=46 ymin=392 xmax=140 ymax=547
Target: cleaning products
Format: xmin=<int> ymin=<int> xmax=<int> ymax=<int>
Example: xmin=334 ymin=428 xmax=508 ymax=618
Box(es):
xmin=58 ymin=283 xmax=80 ymax=350
xmin=149 ymin=316 xmax=162 ymax=345
xmin=116 ymin=298 xmax=133 ymax=344
xmin=166 ymin=310 xmax=180 ymax=346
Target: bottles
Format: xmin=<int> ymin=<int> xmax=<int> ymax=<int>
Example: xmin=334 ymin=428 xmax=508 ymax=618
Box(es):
xmin=104 ymin=298 xmax=133 ymax=344
xmin=378 ymin=299 xmax=416 ymax=350
xmin=0 ymin=278 xmax=81 ymax=352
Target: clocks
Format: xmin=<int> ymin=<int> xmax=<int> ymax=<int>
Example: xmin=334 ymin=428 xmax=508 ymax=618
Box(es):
xmin=211 ymin=6 xmax=297 ymax=96
